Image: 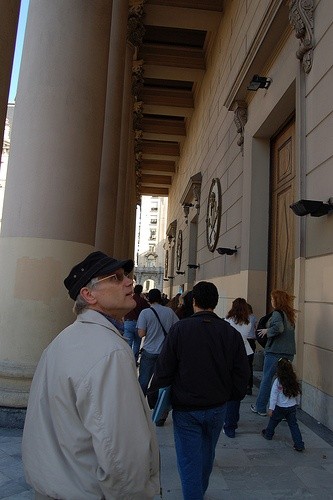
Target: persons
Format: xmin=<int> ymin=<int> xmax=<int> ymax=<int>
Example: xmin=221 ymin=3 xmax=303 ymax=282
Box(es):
xmin=263 ymin=358 xmax=305 ymax=452
xmin=248 ymin=303 xmax=257 ymax=397
xmin=161 ymin=292 xmax=194 ymax=319
xmin=122 ymin=284 xmax=150 ymax=361
xmin=154 ymin=280 xmax=249 ymax=500
xmin=250 ymin=289 xmax=296 ymax=421
xmin=21 ymin=251 xmax=162 ymax=500
xmin=223 ymin=298 xmax=254 ymax=438
xmin=136 ymin=289 xmax=180 ymax=398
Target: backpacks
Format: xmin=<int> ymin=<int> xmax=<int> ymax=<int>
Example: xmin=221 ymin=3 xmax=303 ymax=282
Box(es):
xmin=255 ymin=309 xmax=284 ymax=349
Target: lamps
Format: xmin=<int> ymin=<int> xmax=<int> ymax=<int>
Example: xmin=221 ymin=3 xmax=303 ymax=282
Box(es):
xmin=187 ymin=264 xmax=200 ymax=270
xmin=216 ymin=247 xmax=237 ymax=256
xmin=176 ymin=271 xmax=185 ymax=275
xmin=168 ymin=276 xmax=175 ymax=279
xmin=247 ymin=75 xmax=271 ymax=91
xmin=163 ymin=278 xmax=169 ymax=281
xmin=289 ymin=197 xmax=333 ymax=217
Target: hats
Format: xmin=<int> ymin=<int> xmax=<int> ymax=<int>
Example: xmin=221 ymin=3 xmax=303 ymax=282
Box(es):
xmin=175 ymin=294 xmax=181 ymax=304
xmin=63 ymin=250 xmax=134 ymax=301
xmin=162 ymin=298 xmax=170 ymax=306
xmin=177 ymin=290 xmax=189 ymax=307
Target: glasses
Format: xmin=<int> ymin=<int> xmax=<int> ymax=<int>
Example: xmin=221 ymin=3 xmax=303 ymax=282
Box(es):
xmin=91 ymin=269 xmax=134 ymax=287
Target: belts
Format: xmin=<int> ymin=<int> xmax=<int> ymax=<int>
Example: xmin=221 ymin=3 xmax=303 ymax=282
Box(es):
xmin=125 ymin=319 xmax=133 ymax=321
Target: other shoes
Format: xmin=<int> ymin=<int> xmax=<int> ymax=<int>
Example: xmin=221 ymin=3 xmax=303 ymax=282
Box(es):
xmin=262 ymin=428 xmax=267 ymax=437
xmin=250 ymin=404 xmax=267 ymax=416
xmin=246 ymin=388 xmax=252 ymax=397
xmin=293 ymin=445 xmax=305 ymax=451
